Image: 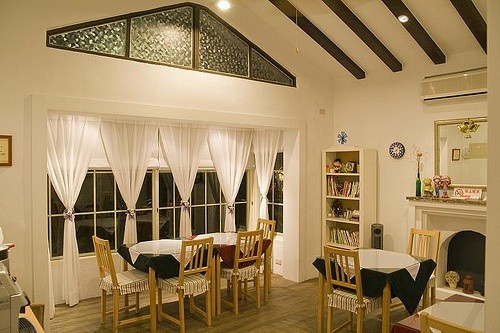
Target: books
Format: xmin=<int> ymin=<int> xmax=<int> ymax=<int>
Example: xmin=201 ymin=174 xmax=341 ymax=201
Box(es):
xmin=327 ymin=175 xmax=360 ymax=198
xmin=330 ymin=224 xmax=359 ymax=248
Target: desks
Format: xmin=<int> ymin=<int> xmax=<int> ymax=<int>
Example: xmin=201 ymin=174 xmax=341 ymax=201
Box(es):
xmin=311 ymin=247 xmax=437 ymax=333
xmin=116 ymin=231 xmax=272 ymax=333
xmin=393 ymin=294 xmax=485 ymax=333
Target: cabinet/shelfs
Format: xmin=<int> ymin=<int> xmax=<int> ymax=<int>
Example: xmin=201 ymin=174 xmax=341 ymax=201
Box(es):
xmin=321 ymin=150 xmax=376 ymax=249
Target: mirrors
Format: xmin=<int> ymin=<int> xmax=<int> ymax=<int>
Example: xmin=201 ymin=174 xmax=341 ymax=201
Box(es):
xmin=434 ymin=116 xmax=488 ymax=189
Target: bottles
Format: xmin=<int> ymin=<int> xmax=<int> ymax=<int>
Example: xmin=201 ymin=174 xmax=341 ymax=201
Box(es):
xmin=463 ymin=275 xmax=474 ymax=294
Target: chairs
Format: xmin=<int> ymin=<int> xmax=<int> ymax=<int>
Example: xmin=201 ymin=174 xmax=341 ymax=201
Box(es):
xmin=220 ymin=229 xmax=264 ymax=316
xmin=323 ymin=245 xmax=382 ymax=333
xmin=158 ymin=236 xmax=214 ymax=333
xmin=92 ymin=235 xmax=156 ymax=333
xmin=255 ymin=218 xmax=276 ymax=293
xmin=407 ymin=228 xmax=441 ymax=309
xmin=420 ymin=311 xmax=482 ymax=333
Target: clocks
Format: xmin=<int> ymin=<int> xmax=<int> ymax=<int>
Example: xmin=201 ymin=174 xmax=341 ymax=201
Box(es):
xmin=389 ymin=142 xmax=405 ymax=158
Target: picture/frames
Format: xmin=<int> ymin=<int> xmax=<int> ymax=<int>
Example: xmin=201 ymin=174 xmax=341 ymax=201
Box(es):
xmin=0 ymin=135 xmax=12 ymax=166
xmin=452 ymin=149 xmax=460 ymax=161
